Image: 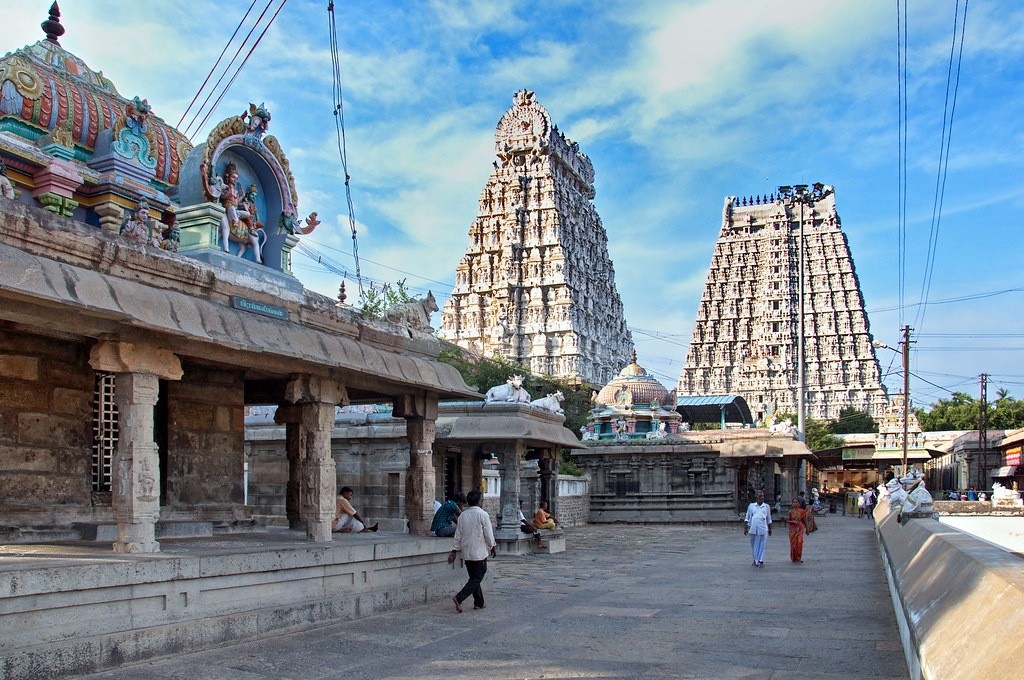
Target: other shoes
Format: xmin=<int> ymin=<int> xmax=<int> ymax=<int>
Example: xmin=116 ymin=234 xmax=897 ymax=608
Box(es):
xmin=474 ymin=603 xmax=486 ymax=609
xmin=453 ymin=597 xmax=462 ymax=613
xmin=754 ymin=560 xmax=763 ymax=567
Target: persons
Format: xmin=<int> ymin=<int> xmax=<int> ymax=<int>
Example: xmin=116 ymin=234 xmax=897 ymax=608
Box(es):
xmin=775 ymin=491 xmax=782 ymax=512
xmin=332 ymin=486 xmax=378 ymax=533
xmin=857 ymin=487 xmax=876 ymax=519
xmin=447 ymin=490 xmax=496 ymax=613
xmin=613 ymin=415 xmax=626 ymax=441
xmin=744 ymin=490 xmax=772 ymax=567
xmin=798 ymin=491 xmax=806 ymax=510
xmin=948 ymin=486 xmax=987 ymax=501
xmin=220 ymin=160 xmax=264 ymax=238
xmin=787 ymin=498 xmax=806 ymax=563
xmin=161 ymin=220 xmax=181 ymax=253
xmin=518 ymin=500 xmax=541 ymax=542
xmin=430 ymin=493 xmax=467 ymax=537
xmin=121 ymin=197 xmax=149 ymax=241
xmin=805 ymin=499 xmax=818 ymax=536
xmin=535 ymin=502 xmax=555 ymax=528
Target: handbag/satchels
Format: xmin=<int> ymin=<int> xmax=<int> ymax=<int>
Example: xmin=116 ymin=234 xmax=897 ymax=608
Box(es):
xmin=871 ymin=492 xmax=875 ymax=503
